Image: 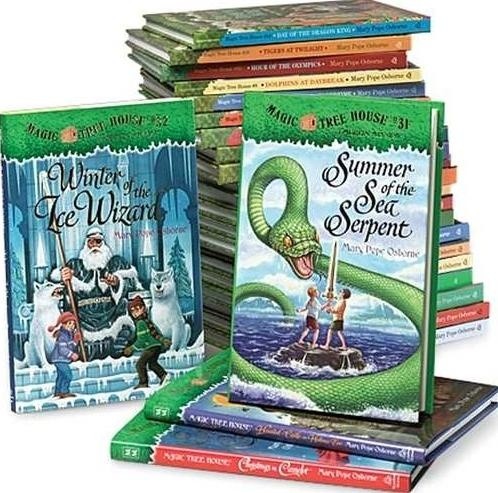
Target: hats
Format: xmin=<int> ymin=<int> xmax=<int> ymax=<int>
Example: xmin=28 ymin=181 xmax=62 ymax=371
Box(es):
xmin=87 ymin=227 xmax=104 ymax=238
xmin=128 ymin=295 xmax=146 ymax=308
xmin=61 ymin=311 xmax=76 ymax=323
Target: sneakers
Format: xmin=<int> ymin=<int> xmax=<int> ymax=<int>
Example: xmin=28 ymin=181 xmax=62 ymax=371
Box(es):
xmin=133 ymin=383 xmax=149 ymax=389
xmin=159 ymin=372 xmax=169 ymax=385
xmin=53 ymin=392 xmax=77 ymax=398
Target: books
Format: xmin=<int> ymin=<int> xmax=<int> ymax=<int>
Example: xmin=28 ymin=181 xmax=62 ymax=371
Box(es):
xmin=144 ymin=346 xmax=498 ymax=468
xmin=109 ymin=409 xmax=434 ymax=493
xmin=227 ymin=89 xmax=446 ymax=427
xmin=0 ymin=95 xmax=207 ymax=413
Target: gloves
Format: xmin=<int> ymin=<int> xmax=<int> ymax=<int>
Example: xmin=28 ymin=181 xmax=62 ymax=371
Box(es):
xmin=74 ymin=332 xmax=81 ymax=341
xmin=124 ymin=349 xmax=131 ymax=356
xmin=162 ymin=339 xmax=172 ymax=347
xmin=71 ymin=353 xmax=79 ymax=361
